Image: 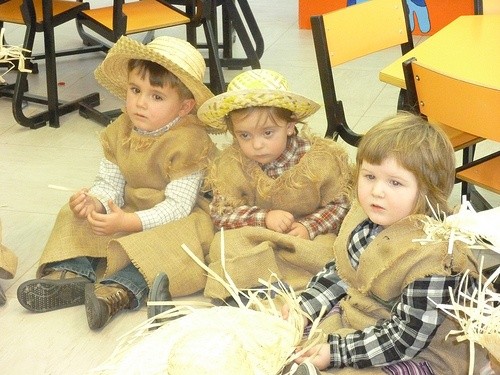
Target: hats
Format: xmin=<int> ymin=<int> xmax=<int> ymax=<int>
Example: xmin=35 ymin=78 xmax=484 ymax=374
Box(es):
xmin=196 ymin=68 xmax=322 ymax=130
xmin=93 ymin=35 xmax=216 ymax=110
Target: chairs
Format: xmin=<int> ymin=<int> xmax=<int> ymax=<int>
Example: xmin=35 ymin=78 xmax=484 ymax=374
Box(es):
xmin=309 ymin=0 xmax=486 ymax=206
xmin=0 ymin=0 xmax=264 ymax=128
xmin=401 ymin=58 xmax=500 ymax=211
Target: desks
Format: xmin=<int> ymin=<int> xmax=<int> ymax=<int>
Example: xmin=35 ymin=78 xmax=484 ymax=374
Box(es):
xmin=380 ymin=14 xmax=500 ymax=124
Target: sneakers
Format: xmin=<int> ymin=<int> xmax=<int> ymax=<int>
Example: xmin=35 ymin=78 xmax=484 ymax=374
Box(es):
xmin=84 ymin=281 xmax=132 ymax=330
xmin=16 ymin=270 xmax=92 ymax=313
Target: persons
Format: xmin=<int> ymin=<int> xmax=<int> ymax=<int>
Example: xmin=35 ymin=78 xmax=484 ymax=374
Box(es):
xmin=18 ymin=35 xmax=215 ymax=329
xmin=196 ymin=68 xmax=353 ymax=307
xmin=146 ymin=114 xmax=495 ymax=374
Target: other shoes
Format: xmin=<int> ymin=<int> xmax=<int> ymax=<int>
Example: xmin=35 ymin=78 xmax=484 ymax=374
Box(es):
xmin=145 ymin=271 xmax=188 ymax=331
